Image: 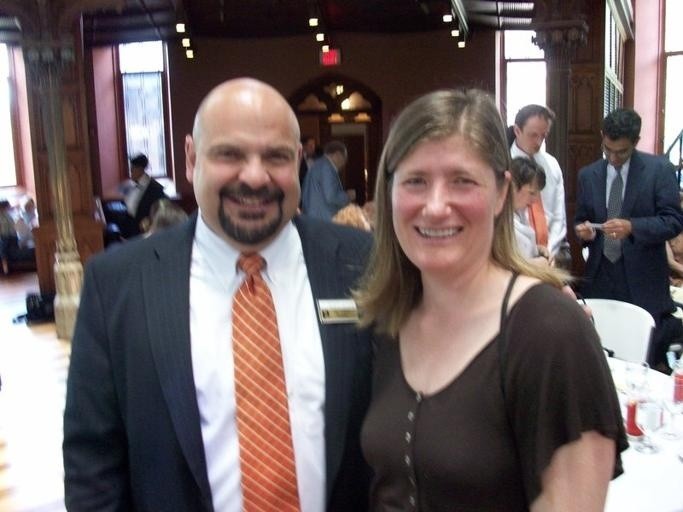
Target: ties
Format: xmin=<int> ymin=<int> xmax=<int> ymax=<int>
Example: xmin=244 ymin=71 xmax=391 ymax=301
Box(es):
xmin=527 ymin=155 xmax=548 ymax=246
xmin=232 ymin=251 xmax=301 ymax=512
xmin=603 ymin=166 xmax=623 ymax=263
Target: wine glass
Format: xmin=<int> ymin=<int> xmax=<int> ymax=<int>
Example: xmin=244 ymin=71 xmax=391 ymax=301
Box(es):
xmin=666 ymin=343 xmax=683 ymax=413
xmin=612 ymin=359 xmax=664 ymax=454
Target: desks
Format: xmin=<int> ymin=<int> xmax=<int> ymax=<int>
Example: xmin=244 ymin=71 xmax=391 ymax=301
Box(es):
xmin=598 ymin=356 xmax=683 ymax=511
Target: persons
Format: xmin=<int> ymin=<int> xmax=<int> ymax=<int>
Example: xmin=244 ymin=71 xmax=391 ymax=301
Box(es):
xmin=17 ymin=196 xmax=39 ymax=249
xmin=0 ymin=201 xmax=18 ymax=275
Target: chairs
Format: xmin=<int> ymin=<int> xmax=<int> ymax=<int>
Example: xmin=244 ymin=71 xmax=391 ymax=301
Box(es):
xmin=575 ymin=297 xmax=658 ymax=367
